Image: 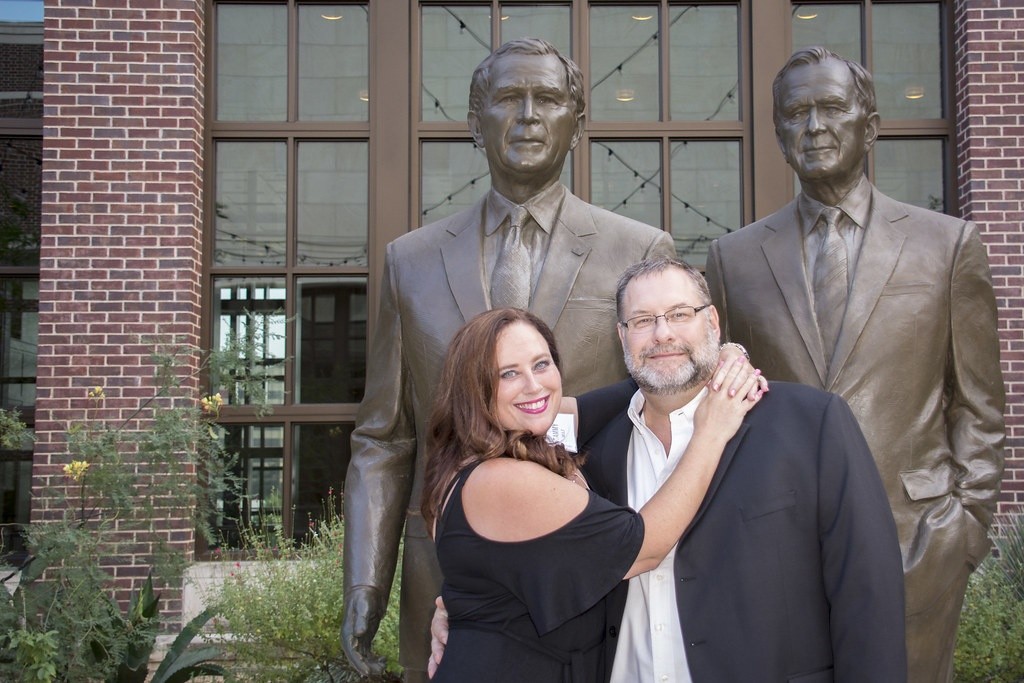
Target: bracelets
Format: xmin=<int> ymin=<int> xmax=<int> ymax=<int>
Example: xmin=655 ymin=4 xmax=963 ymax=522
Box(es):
xmin=719 ymin=342 xmax=751 ymax=361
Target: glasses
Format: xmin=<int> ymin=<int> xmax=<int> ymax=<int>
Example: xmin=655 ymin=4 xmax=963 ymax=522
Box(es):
xmin=620 ymin=304 xmax=712 ymax=335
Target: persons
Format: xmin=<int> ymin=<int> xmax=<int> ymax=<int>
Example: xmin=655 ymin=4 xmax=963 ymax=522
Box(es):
xmin=425 ymin=253 xmax=910 ymax=683
xmin=421 ymin=307 xmax=771 ymax=683
xmin=704 ymin=47 xmax=1007 ymax=683
xmin=339 ymin=37 xmax=680 ymax=683
